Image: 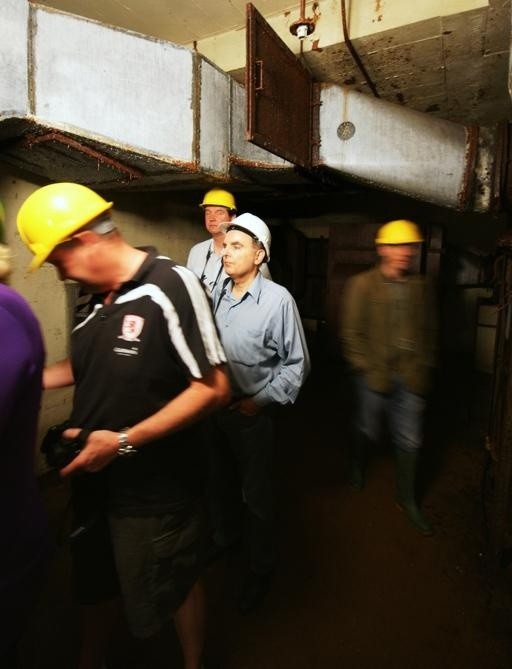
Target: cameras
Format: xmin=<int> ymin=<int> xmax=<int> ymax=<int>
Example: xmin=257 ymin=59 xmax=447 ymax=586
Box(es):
xmin=40 ymin=421 xmax=89 ymax=455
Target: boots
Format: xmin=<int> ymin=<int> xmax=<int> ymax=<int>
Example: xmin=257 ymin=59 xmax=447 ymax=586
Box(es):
xmin=389 ymin=442 xmax=435 ymax=537
xmin=345 ymin=430 xmax=380 ymax=489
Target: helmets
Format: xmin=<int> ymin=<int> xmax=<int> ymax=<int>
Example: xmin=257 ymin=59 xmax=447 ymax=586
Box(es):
xmin=374 ymin=219 xmax=425 ymax=246
xmin=217 ymin=212 xmax=272 ymax=263
xmin=198 ymin=187 xmax=238 ymax=211
xmin=15 ymin=181 xmax=115 ymax=274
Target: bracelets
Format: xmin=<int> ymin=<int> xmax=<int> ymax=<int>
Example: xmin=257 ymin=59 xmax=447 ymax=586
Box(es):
xmin=117 ymin=429 xmax=136 ymax=458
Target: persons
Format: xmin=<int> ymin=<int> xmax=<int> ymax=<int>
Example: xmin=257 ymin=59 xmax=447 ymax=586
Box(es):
xmin=196 ymin=211 xmax=312 ymax=618
xmin=336 ymin=219 xmax=441 ymax=537
xmin=185 ymin=188 xmax=273 ymax=309
xmin=1 ymin=230 xmax=56 ymax=668
xmin=18 ymin=180 xmax=234 ymax=669
